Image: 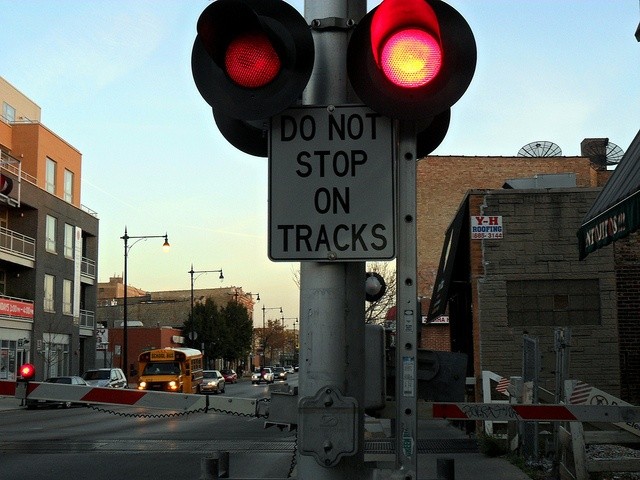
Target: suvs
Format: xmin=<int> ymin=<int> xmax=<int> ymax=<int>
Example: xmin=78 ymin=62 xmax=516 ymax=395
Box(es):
xmin=81 ymin=368 xmax=127 ymax=389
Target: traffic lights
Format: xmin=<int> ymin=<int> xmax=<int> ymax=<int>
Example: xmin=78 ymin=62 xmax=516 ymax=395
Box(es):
xmin=191 ymin=0 xmax=315 ymax=121
xmin=211 ymin=107 xmax=268 ymax=158
xmin=347 ymin=0 xmax=477 ymax=120
xmin=417 ymin=109 xmax=451 ymax=160
xmin=19 ymin=363 xmax=35 ymax=381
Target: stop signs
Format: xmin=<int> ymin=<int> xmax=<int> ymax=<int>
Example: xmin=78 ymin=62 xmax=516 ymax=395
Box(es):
xmin=267 ymin=104 xmax=399 ymax=262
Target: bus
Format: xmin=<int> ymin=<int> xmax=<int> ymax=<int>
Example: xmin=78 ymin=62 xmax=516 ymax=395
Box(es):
xmin=137 ymin=346 xmax=203 ymax=393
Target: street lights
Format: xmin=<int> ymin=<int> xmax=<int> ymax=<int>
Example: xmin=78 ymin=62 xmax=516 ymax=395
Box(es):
xmin=188 ymin=264 xmax=224 ymax=348
xmin=229 ymin=289 xmax=260 ymax=378
xmin=261 ymin=304 xmax=283 ymax=367
xmin=280 ymin=315 xmax=298 ymax=367
xmin=120 ymin=225 xmax=170 ymax=386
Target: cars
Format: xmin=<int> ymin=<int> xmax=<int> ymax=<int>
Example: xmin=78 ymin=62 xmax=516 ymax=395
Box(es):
xmin=252 ymin=368 xmax=274 ymax=384
xmin=284 ymin=365 xmax=294 ymax=374
xmin=220 ymin=370 xmax=237 ymax=384
xmin=203 ymin=370 xmax=225 ymax=395
xmin=273 ymin=367 xmax=287 ymax=380
xmin=26 ymin=376 xmax=90 ymax=410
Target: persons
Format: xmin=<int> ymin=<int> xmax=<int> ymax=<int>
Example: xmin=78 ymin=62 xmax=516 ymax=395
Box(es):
xmin=257 ymin=367 xmax=269 ymax=384
